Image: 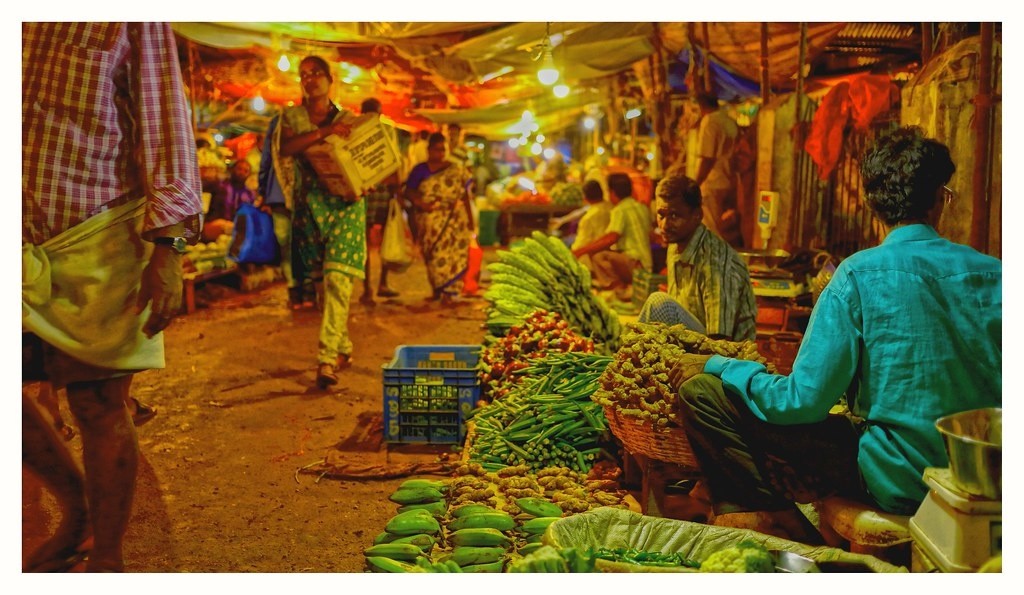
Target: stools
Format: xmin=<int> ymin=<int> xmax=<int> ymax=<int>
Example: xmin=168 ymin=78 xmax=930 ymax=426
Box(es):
xmin=815 ymin=497 xmax=913 ymax=555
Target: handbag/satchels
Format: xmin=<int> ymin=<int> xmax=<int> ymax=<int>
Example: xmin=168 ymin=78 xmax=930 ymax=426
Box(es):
xmin=227 ymin=196 xmax=283 ymax=266
xmin=380 ymin=197 xmax=416 ymax=274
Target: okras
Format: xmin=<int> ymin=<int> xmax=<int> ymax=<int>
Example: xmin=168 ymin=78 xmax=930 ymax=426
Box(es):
xmin=465 ymin=353 xmax=615 ymax=472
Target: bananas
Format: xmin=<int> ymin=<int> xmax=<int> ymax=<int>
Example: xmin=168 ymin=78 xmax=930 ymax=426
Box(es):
xmin=363 ymin=480 xmax=566 ymax=573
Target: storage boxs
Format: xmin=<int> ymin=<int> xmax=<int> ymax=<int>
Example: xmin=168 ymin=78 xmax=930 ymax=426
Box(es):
xmin=379 ymin=344 xmax=484 ymax=444
xmin=480 ymin=210 xmax=498 ymax=246
xmin=305 ymin=110 xmax=401 ymax=198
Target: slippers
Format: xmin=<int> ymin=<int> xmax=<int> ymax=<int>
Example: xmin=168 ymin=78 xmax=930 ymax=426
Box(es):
xmin=22 ymin=537 xmax=124 ymax=573
xmin=56 ymin=422 xmax=78 ymax=441
xmin=127 ymin=395 xmax=157 ymax=423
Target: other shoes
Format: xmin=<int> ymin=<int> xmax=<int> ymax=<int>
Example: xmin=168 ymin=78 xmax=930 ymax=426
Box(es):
xmin=287 ymin=285 xmax=302 ymax=310
xmin=301 ymin=282 xmax=315 ymax=308
xmin=317 ymin=362 xmax=339 ymax=385
xmin=332 ymin=353 xmax=353 ymax=372
xmin=378 ymin=283 xmax=401 ymax=298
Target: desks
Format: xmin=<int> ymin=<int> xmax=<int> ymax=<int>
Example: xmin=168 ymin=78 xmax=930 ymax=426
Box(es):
xmin=501 ymin=204 xmax=578 ymax=242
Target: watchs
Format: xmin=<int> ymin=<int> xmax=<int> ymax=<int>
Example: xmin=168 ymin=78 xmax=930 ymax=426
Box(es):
xmin=153 ymin=236 xmax=187 ymax=254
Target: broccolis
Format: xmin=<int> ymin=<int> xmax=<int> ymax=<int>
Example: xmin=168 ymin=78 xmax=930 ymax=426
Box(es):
xmin=699 ymin=541 xmax=773 ymax=573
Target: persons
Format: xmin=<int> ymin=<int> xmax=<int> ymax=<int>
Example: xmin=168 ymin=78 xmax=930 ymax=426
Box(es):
xmin=571 ymin=173 xmax=654 ymax=292
xmin=277 ymin=55 xmax=369 ymax=386
xmin=403 ymin=132 xmax=475 ymax=310
xmin=570 ymin=180 xmax=614 ymax=253
xmin=359 ymin=97 xmax=400 ymax=302
xmin=694 ymin=91 xmax=737 ymax=242
xmin=667 ymin=125 xmax=1003 ymax=547
xmin=397 ymin=123 xmax=469 ymax=263
xmin=22 ymin=22 xmax=204 ymax=573
xmin=196 ymin=114 xmax=324 ymax=311
xmin=637 ymin=173 xmax=757 ymax=343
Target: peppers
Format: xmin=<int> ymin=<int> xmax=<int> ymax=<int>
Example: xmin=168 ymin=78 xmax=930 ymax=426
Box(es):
xmin=474 ymin=307 xmax=595 ymax=403
xmin=588 ymin=544 xmax=702 ymax=568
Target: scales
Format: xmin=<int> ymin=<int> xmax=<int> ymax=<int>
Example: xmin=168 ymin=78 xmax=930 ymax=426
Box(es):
xmin=749 ymin=268 xmax=797 ymax=298
xmin=907 ymin=466 xmax=1002 ymax=573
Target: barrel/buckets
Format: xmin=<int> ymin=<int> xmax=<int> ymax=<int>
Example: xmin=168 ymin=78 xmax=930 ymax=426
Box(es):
xmin=477 ymin=211 xmax=501 ymax=245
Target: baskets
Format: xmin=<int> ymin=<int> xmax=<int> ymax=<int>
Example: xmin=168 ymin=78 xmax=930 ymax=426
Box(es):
xmin=600 ymin=402 xmax=703 ymax=472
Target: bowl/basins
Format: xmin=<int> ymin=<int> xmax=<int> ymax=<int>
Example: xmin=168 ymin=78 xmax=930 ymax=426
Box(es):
xmin=936 ymin=407 xmax=1001 ymax=501
xmin=737 ymin=250 xmax=790 ymax=273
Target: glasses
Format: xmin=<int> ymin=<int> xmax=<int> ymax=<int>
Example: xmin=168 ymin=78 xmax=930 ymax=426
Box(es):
xmin=299 ymin=67 xmax=326 ymax=82
